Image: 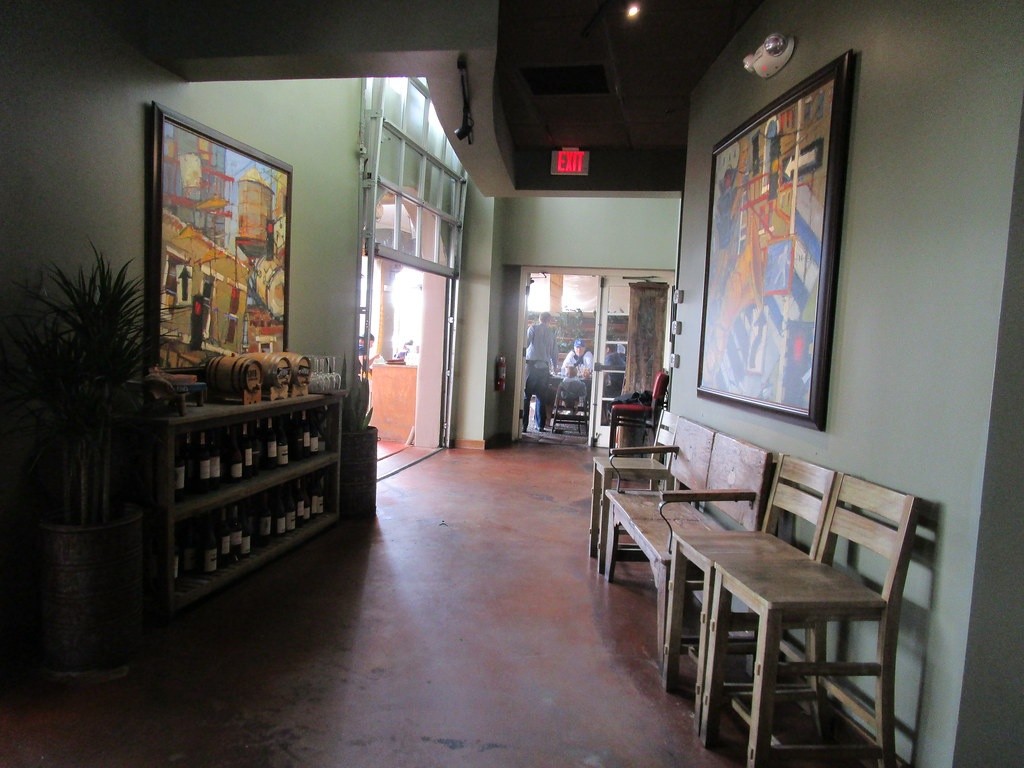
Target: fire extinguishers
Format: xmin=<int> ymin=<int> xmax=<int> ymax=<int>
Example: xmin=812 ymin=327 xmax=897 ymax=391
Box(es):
xmin=496 ymin=352 xmax=507 ymax=392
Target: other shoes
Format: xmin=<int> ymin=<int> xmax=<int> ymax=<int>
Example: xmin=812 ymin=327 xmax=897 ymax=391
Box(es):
xmin=537 ymin=427 xmax=543 ymax=431
xmin=522 ymin=427 xmax=526 ymax=432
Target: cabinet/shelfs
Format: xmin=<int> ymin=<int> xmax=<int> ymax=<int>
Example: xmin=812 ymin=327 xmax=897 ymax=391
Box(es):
xmin=111 ymin=392 xmax=344 ymax=623
xmin=371 ymin=363 xmax=417 ymax=442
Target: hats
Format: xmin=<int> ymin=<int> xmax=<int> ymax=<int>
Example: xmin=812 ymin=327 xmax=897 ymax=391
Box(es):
xmin=359 ymin=332 xmax=375 ymax=340
xmin=574 ymin=339 xmax=586 ymax=347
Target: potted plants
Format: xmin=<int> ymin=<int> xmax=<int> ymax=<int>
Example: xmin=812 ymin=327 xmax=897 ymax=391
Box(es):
xmin=325 ymin=351 xmax=378 ymax=521
xmin=0 ymin=233 xmax=175 ymax=684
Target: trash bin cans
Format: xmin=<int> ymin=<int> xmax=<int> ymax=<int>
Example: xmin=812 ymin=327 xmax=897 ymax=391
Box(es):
xmin=618 ymin=417 xmax=652 ymax=458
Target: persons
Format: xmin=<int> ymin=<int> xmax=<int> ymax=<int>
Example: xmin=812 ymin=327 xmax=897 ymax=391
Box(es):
xmin=561 ymin=338 xmax=626 ymax=426
xmin=522 ymin=313 xmax=557 ymax=432
xmin=358 ymin=333 xmax=380 ymax=378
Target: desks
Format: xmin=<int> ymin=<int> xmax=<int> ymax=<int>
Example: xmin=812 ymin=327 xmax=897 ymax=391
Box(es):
xmin=548 ymin=375 xmax=592 ymax=401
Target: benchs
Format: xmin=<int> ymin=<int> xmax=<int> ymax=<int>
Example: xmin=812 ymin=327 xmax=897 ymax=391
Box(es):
xmin=605 ymin=415 xmax=774 ymax=673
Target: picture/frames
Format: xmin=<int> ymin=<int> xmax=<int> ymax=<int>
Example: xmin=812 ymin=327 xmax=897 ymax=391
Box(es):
xmin=696 ymin=49 xmax=856 ymax=433
xmin=144 ymin=100 xmax=294 ymax=383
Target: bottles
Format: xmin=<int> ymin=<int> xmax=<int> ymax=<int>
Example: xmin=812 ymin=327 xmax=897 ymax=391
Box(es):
xmin=174 ymin=405 xmax=326 ymax=582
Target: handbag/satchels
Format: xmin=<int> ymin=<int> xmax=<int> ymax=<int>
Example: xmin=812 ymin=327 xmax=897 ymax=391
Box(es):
xmin=612 ymin=392 xmax=639 ymax=403
xmin=638 ymin=390 xmax=652 ymax=406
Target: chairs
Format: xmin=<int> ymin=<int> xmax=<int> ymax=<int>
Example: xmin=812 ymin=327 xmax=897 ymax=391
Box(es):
xmin=700 ymin=471 xmax=922 ymax=768
xmin=552 ymin=379 xmax=588 ymax=437
xmin=660 ymin=452 xmax=837 ymax=738
xmin=588 ymin=409 xmax=680 ymax=574
xmin=608 ymin=371 xmax=669 ymax=479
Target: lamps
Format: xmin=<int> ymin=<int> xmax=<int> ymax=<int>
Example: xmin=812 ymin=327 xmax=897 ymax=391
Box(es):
xmin=742 ymin=33 xmax=795 ymax=80
